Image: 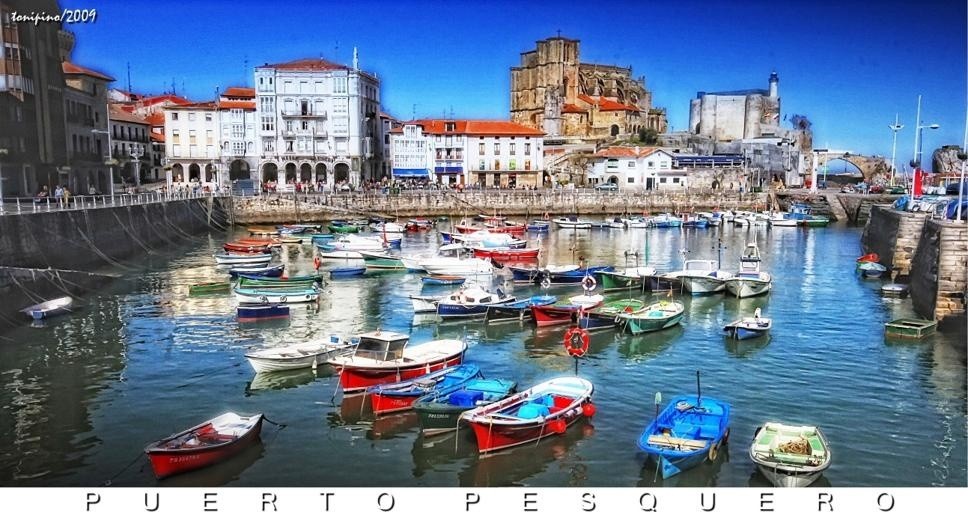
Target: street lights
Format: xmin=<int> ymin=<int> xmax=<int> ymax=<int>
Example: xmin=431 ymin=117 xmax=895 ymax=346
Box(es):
xmin=909 ymin=94 xmax=939 ymax=203
xmin=91 ymin=129 xmax=114 ymax=209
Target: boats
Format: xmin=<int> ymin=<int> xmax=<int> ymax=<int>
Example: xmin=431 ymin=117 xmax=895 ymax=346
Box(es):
xmin=747 ymin=423 xmax=830 ymax=488
xmin=636 ymin=369 xmax=729 ymax=481
xmin=855 ymin=253 xmax=880 ymax=262
xmin=856 ymin=261 xmax=886 ymax=278
xmin=23 ymin=296 xmax=73 ymax=320
xmin=145 ymin=412 xmax=263 ymax=479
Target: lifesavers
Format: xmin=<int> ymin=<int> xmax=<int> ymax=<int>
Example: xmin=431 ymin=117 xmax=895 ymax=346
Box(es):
xmin=564 ymin=328 xmax=590 ymax=355
xmin=582 ymin=277 xmax=597 ymax=291
xmin=542 ymin=278 xmax=551 ymax=288
xmin=313 ymin=256 xmax=321 ymax=270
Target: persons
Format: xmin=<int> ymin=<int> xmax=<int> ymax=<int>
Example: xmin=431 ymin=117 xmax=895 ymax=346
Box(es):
xmin=54 ymin=184 xmax=72 ymax=209
xmin=122 ymin=180 xmax=144 ymax=198
xmin=90 ymin=184 xmax=96 ymax=200
xmin=175 ymin=182 xmax=219 ymax=198
xmin=263 ymin=178 xmax=464 ymax=195
